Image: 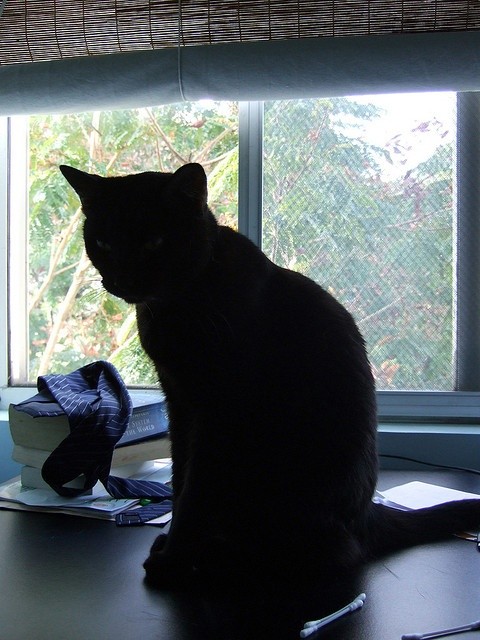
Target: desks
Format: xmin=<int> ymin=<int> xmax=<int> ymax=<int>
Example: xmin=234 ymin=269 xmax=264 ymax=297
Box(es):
xmin=0 ymin=471 xmax=480 ymax=639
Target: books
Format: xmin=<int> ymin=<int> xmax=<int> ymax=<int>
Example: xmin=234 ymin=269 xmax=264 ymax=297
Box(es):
xmin=11 ymin=432 xmax=172 ymax=469
xmin=8 ymin=395 xmax=170 ymax=450
xmin=18 ymin=459 xmax=176 ymax=495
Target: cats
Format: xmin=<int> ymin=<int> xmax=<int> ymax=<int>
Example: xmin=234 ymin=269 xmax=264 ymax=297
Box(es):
xmin=58 ymin=163 xmax=377 ymax=640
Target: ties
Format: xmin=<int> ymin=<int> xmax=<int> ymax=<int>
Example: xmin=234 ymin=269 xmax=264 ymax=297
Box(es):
xmin=15 ymin=360 xmax=172 ymax=528
